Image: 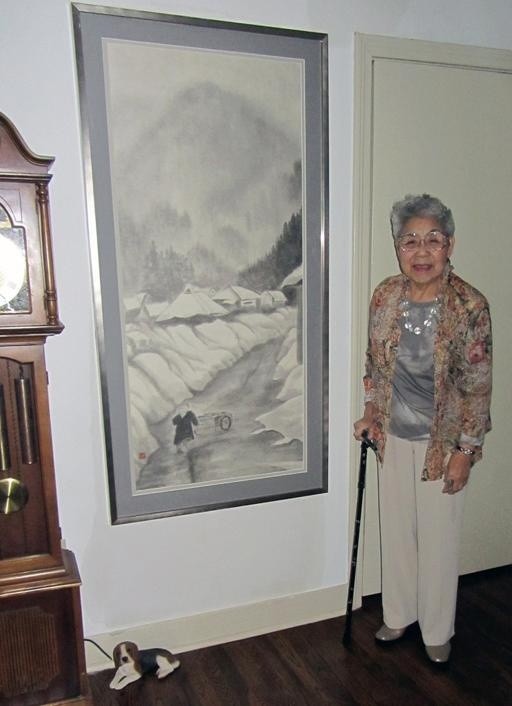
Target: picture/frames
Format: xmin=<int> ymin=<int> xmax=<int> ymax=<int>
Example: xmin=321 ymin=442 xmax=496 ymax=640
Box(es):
xmin=67 ymin=0 xmax=334 ymax=528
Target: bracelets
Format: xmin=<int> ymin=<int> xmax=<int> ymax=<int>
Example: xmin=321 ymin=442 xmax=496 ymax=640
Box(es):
xmin=455 ymin=444 xmax=477 ymax=456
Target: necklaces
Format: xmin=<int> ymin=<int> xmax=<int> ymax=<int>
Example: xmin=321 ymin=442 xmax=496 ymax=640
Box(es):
xmin=401 ymin=262 xmax=452 ymax=334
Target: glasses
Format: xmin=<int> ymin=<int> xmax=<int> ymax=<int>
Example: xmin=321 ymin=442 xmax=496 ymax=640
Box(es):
xmin=397 ymin=230 xmax=450 ymax=252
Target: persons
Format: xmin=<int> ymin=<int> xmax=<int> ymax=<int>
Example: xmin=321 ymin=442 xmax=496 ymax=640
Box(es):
xmin=353 ymin=196 xmax=491 ymax=670
xmin=169 ymin=403 xmax=201 ymax=454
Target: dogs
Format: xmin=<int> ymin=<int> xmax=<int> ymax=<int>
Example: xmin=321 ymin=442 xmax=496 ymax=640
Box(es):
xmin=109 ymin=642 xmax=180 ymax=690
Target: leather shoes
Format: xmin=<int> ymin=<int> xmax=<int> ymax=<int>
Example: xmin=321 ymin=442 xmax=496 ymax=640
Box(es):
xmin=425 ymin=642 xmax=452 ymax=663
xmin=376 ymin=623 xmax=406 ymax=641
